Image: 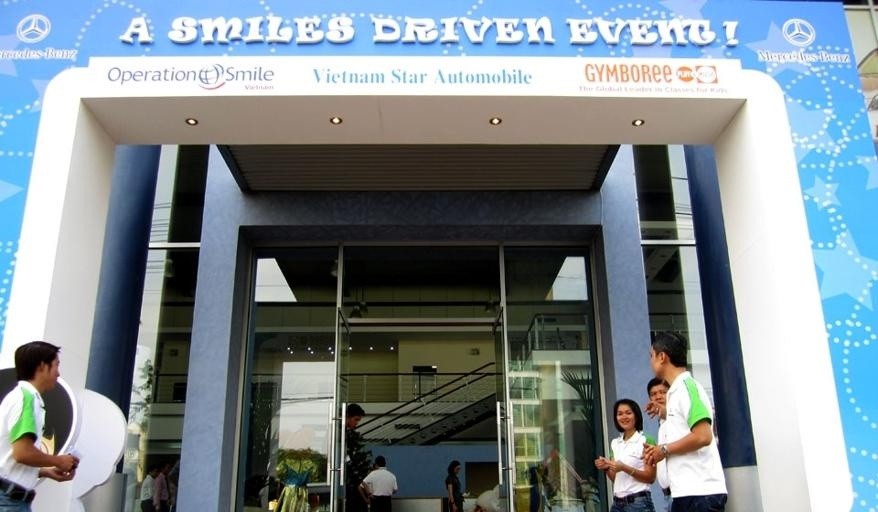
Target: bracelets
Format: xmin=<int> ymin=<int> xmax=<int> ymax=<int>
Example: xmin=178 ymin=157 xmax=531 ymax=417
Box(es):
xmin=450 ymin=501 xmax=454 ymax=505
xmin=627 ymin=468 xmax=634 ymax=476
xmin=603 ymin=467 xmax=610 ymax=472
xmin=659 ymin=444 xmax=672 ymax=463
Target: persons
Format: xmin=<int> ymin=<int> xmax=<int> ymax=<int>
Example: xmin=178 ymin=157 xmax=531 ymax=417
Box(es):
xmin=166 ymin=455 xmax=180 ymax=512
xmin=139 ymin=463 xmax=158 ymax=512
xmin=593 ymin=399 xmax=656 ymax=511
xmin=639 ymin=330 xmax=728 ymax=512
xmin=334 ymin=403 xmax=367 ymax=512
xmin=0 ymin=341 xmax=79 ymax=512
xmin=642 ymin=376 xmax=674 ymax=512
xmin=444 ymin=460 xmax=464 ymax=512
xmin=357 ymin=464 xmax=378 ymax=512
xmin=152 ymin=459 xmax=175 ymax=512
xmin=361 ymin=455 xmax=398 ymax=512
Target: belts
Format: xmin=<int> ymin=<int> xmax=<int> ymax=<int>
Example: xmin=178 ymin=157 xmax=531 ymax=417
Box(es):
xmin=0 ymin=476 xmax=36 ymax=504
xmin=613 ymin=490 xmax=652 ymax=503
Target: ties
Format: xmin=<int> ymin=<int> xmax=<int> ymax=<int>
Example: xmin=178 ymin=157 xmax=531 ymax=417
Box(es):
xmin=165 ymin=476 xmax=171 ymax=500
